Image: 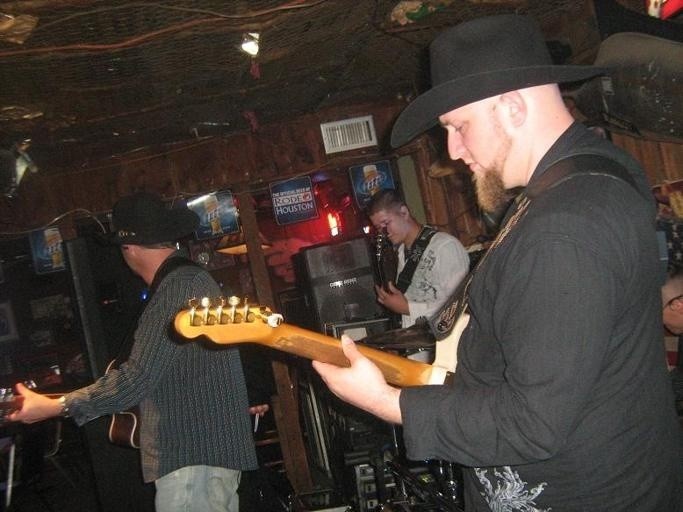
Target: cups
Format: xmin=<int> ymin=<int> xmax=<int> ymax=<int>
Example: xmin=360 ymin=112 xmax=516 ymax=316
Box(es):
xmin=43 ymin=228 xmax=64 ymax=269
xmin=202 ymin=196 xmax=223 ymax=235
xmin=361 ymin=165 xmax=379 ymax=196
xmin=344 ymin=303 xmax=362 ymax=320
xmin=667 ymin=191 xmax=683 ymax=217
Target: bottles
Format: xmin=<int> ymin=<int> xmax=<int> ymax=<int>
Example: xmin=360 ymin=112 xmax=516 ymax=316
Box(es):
xmin=0 ymin=379 xmax=36 ymax=400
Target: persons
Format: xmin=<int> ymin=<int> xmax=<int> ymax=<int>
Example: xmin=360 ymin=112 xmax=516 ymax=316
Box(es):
xmin=367 ymin=190 xmax=471 ymax=325
xmin=4 ymin=194 xmax=258 ymax=512
xmin=313 ymin=12 xmax=683 ymax=512
xmin=661 ymin=276 xmax=682 ymax=418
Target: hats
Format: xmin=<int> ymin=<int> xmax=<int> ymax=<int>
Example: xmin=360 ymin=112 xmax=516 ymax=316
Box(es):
xmin=92 ymin=192 xmax=200 ymax=246
xmin=390 ymin=15 xmax=609 ymax=150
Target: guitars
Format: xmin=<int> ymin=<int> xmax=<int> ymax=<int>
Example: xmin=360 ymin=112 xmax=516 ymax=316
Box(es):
xmin=175 ymin=294 xmax=471 ymax=422
xmin=1 ymin=358 xmax=140 ymax=449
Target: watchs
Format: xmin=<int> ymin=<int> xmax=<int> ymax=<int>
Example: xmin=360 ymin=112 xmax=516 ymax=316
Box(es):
xmin=59 ymin=397 xmax=72 ymax=420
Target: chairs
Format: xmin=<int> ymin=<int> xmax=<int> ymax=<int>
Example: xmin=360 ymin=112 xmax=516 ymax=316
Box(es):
xmin=41 ymin=420 xmax=78 ymax=489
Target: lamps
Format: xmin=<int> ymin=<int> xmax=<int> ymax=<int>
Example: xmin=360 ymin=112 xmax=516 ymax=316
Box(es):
xmin=241 ymin=31 xmax=260 ymax=55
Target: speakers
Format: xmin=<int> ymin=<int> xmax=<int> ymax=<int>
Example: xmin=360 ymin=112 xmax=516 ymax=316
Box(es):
xmin=299 ymin=235 xmax=390 ymax=334
